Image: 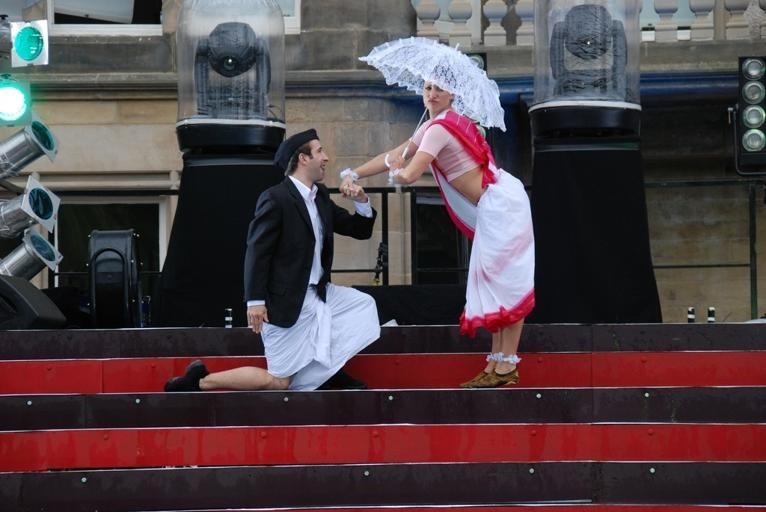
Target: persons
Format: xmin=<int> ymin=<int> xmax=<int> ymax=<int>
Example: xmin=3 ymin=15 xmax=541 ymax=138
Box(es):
xmin=159 ymin=128 xmax=379 ymax=393
xmin=336 ymin=66 xmax=539 ymax=389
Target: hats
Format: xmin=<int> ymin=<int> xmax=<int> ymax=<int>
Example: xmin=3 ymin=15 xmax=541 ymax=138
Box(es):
xmin=272 ymin=128 xmax=319 ymax=177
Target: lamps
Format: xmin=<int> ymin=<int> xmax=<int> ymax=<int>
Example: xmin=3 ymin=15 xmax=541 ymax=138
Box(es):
xmin=0 ymin=19 xmax=65 ymax=278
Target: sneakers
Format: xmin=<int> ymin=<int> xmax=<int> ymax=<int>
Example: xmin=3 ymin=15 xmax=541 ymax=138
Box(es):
xmin=459 ymin=364 xmax=520 ymax=389
xmin=164 ymin=359 xmax=211 ymax=392
xmin=315 ymin=367 xmax=369 ymax=390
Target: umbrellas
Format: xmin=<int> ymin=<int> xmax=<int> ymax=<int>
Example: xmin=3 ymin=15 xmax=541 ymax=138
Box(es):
xmin=356 ymin=35 xmax=507 ymax=169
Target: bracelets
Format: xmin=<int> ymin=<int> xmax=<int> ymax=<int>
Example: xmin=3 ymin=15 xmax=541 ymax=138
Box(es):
xmin=339 ymin=168 xmax=359 ymax=181
xmin=387 ymin=168 xmax=403 ymax=187
